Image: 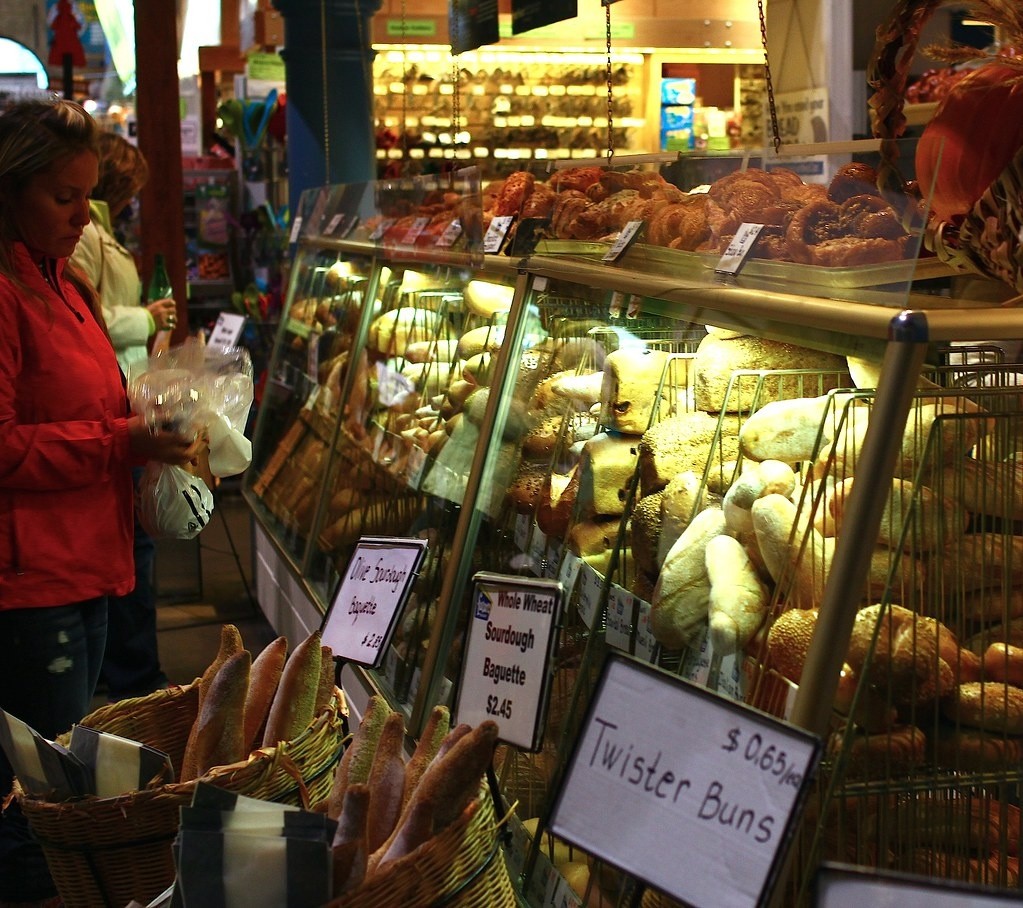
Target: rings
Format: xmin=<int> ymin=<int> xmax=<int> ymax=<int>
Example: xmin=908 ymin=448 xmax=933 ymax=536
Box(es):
xmin=170 ymin=314 xmax=173 ymax=322
xmin=169 ymin=323 xmax=172 ymax=328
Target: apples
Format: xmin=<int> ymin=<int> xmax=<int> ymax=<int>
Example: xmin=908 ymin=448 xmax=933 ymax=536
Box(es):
xmin=903 ymin=65 xmax=977 ymax=106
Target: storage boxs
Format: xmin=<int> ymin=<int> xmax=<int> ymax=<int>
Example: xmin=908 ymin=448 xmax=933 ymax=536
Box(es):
xmin=661 ymin=105 xmax=693 ymax=127
xmin=661 ymin=77 xmax=697 ymax=104
xmin=661 ymin=127 xmax=694 ymax=151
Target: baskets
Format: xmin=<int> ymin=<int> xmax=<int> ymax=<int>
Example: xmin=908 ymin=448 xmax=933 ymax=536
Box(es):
xmin=863 ymin=0 xmax=1023 ymax=294
xmin=10 ymin=676 xmax=518 ymax=907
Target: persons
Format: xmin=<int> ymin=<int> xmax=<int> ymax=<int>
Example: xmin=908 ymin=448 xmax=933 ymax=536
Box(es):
xmin=68 ymin=132 xmax=178 ymax=379
xmin=0 ymin=99 xmax=208 ymax=896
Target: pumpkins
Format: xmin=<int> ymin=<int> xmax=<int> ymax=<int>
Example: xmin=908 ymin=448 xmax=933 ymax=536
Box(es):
xmin=913 ymin=46 xmax=1023 ymax=218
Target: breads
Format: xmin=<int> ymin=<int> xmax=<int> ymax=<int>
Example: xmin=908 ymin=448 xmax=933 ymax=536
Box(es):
xmin=177 ymin=623 xmax=498 ymax=885
xmin=252 ymin=168 xmax=1023 ymax=895
